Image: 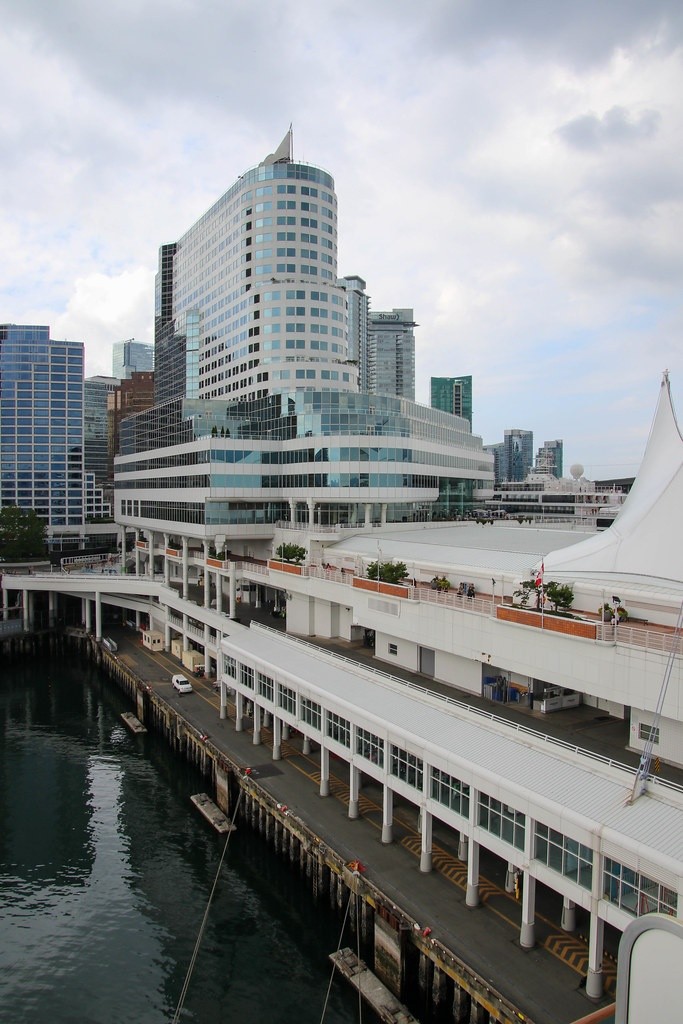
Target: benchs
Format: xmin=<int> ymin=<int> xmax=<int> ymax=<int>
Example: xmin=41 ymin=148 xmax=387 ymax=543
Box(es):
xmin=626 ymin=617 xmax=648 ymax=626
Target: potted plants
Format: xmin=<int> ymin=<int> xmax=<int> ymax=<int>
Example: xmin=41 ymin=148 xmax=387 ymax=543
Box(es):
xmin=496 ymin=579 xmax=605 ymax=641
xmin=266 ymin=543 xmax=309 ymax=575
xmin=207 ymin=546 xmax=231 ymax=568
xmin=136 ymin=535 xmax=149 ymax=549
xmin=166 ymin=538 xmax=183 ymax=557
xmin=352 ymin=562 xmax=410 ymax=599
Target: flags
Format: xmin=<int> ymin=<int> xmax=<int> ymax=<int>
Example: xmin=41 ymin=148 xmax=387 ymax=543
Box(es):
xmin=534 ymin=563 xmax=544 ymax=587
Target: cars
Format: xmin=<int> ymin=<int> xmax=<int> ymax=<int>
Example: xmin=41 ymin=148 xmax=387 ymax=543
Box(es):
xmin=171 ymin=674 xmax=193 ymax=695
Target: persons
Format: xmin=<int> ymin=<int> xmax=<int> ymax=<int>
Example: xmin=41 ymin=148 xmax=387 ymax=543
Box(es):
xmin=457 ymin=582 xmax=476 ymax=600
xmin=323 ymin=563 xmax=331 ymax=569
xmin=411 ymin=578 xmax=417 ymax=587
xmin=539 ymin=595 xmax=546 ymax=608
xmin=611 ymin=614 xmax=616 ymax=635
xmin=341 ymin=568 xmax=345 ymax=573
